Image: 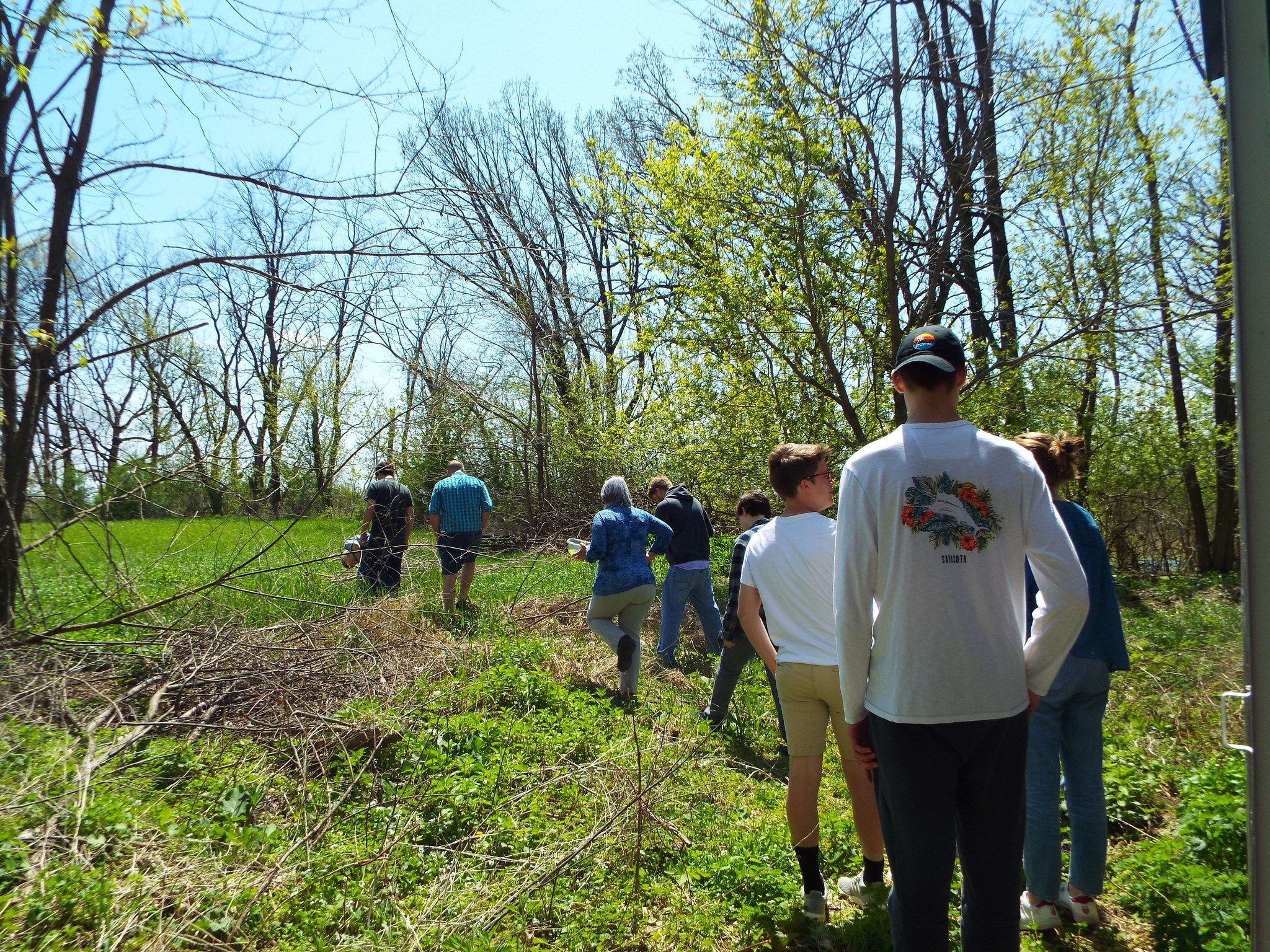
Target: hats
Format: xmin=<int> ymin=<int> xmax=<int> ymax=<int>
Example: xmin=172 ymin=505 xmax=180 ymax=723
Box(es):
xmin=892 ymin=325 xmax=965 ymax=374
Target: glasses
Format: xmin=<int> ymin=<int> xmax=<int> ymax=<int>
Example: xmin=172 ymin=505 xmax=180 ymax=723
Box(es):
xmin=797 ymin=471 xmax=832 ymax=486
xmin=736 ymin=516 xmax=740 ymax=525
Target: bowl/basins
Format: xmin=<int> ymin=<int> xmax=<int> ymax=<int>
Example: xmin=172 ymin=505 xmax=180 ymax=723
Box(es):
xmin=567 ymin=538 xmax=591 ymax=554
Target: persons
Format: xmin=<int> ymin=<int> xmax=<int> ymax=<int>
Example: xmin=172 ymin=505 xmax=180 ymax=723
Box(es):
xmin=569 ymin=475 xmax=674 ymax=705
xmin=832 ymin=323 xmax=1091 ymax=952
xmin=341 ymin=533 xmax=371 ymax=569
xmin=1012 ymin=430 xmax=1132 ymax=932
xmin=736 ymin=440 xmax=889 ymax=922
xmin=646 ymin=476 xmax=724 ymax=668
xmin=356 ymin=461 xmax=415 ymax=597
xmin=427 ymin=459 xmax=493 ymax=624
xmin=702 ymin=491 xmax=790 ymax=755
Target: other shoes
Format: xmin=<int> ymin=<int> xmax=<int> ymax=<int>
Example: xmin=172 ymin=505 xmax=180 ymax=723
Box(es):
xmin=617 ymin=635 xmax=636 ymax=673
xmin=1058 ymin=881 xmax=1099 ymax=925
xmin=695 ymin=711 xmax=721 ymax=731
xmin=838 ymin=867 xmax=885 ymax=912
xmin=801 ymin=873 xmax=828 ymax=922
xmin=1020 ymin=889 xmax=1061 ymax=930
